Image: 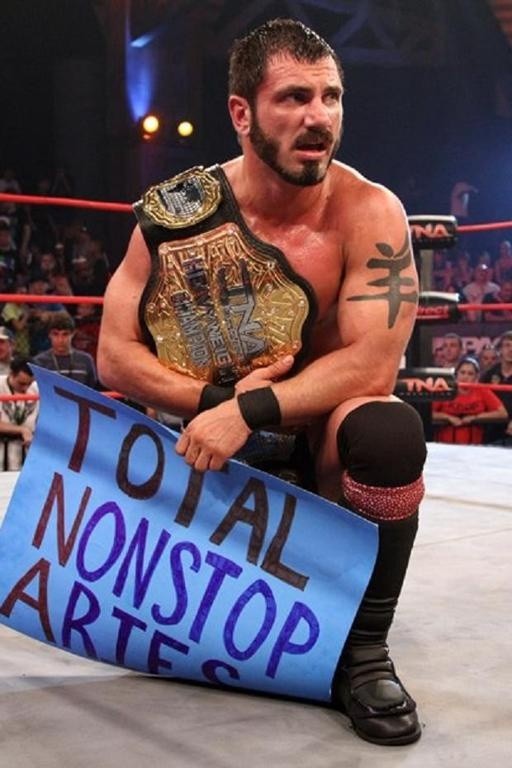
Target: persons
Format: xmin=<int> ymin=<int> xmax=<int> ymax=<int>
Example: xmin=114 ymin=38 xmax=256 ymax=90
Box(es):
xmin=1 ymin=166 xmax=110 ymax=474
xmin=96 ymin=18 xmax=427 ymax=746
xmin=392 ymin=240 xmax=511 ymax=446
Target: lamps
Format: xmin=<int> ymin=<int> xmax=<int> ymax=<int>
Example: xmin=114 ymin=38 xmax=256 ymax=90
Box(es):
xmin=134 ymin=115 xmax=198 ymax=148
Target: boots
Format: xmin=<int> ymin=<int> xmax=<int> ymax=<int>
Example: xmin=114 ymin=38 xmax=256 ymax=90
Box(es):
xmin=335 ymin=596 xmax=422 ymax=746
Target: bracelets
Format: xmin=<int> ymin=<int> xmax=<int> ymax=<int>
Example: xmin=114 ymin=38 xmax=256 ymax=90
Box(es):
xmin=236 ymin=386 xmax=282 ymax=432
xmin=197 ymin=383 xmax=235 ymax=414
